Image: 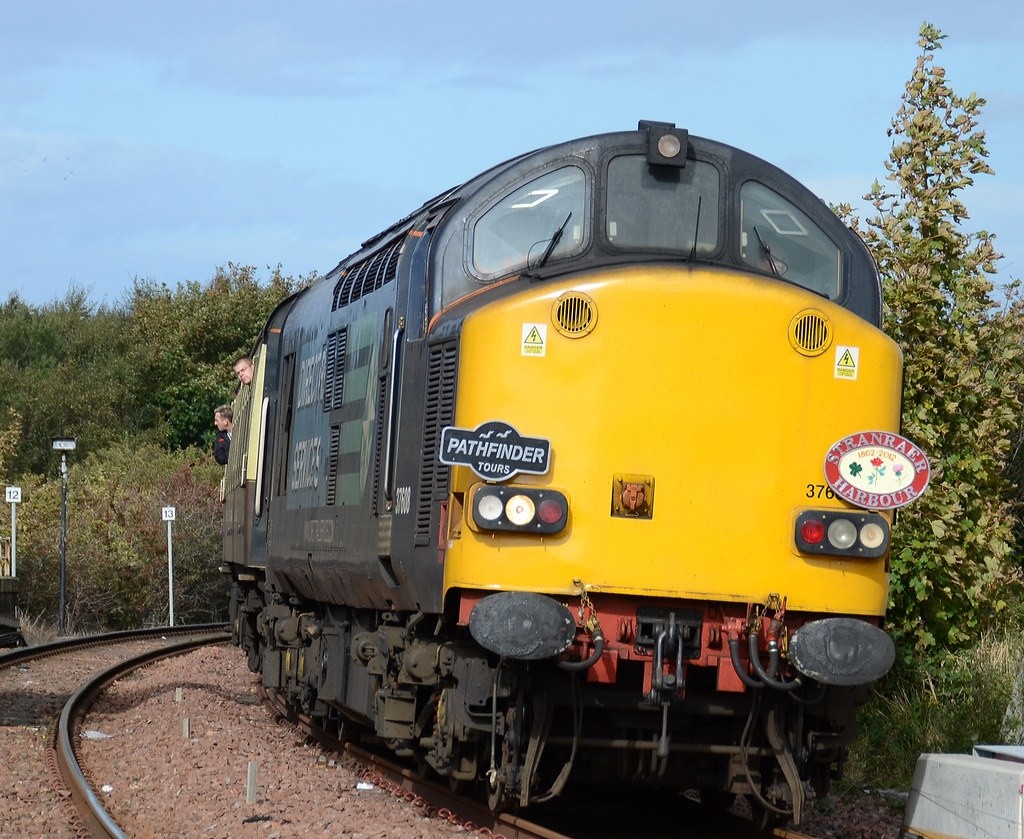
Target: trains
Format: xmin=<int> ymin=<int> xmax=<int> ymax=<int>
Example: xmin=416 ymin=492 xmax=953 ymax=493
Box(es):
xmin=219 ymin=121 xmax=932 ymax=812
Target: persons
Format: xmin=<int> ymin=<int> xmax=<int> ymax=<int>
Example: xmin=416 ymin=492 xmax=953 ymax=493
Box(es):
xmin=212 ymin=403 xmax=233 ymax=466
xmin=233 ymin=355 xmax=255 ymax=385
